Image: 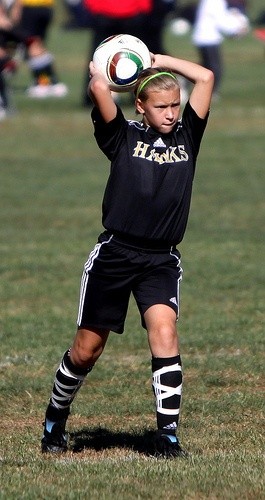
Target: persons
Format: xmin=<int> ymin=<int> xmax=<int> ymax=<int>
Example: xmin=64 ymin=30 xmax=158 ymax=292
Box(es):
xmin=41 ymin=52 xmax=216 ymax=459
xmin=0 ymin=0 xmax=265 ymax=113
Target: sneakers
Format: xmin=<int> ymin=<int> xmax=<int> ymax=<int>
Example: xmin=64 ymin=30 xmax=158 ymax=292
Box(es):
xmin=40 ymin=433 xmax=69 ymax=454
xmin=151 ymin=430 xmax=182 ymax=458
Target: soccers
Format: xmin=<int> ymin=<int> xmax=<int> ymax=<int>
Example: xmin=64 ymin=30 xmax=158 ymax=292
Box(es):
xmin=93 ymin=33 xmax=151 ymax=93
